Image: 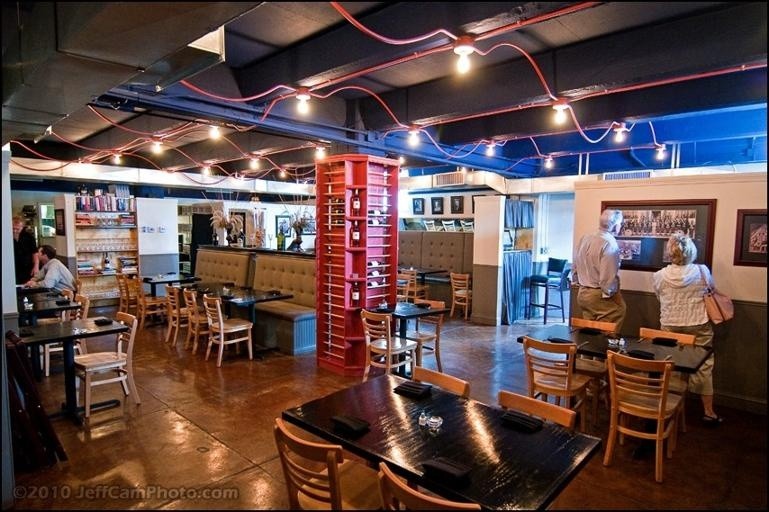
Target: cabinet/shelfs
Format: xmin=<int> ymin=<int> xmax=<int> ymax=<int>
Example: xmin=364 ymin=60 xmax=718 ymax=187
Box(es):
xmin=72 ymin=195 xmax=140 ymax=311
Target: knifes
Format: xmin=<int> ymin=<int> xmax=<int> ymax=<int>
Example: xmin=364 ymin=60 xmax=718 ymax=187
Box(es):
xmin=569 ymin=325 xmax=687 ymax=362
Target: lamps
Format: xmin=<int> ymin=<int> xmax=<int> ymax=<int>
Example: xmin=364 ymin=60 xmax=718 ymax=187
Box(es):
xmin=7 ymin=0 xmax=670 ymax=191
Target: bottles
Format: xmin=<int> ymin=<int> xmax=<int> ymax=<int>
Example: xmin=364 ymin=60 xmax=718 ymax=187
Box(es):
xmin=212 ymin=228 xmax=219 ymax=246
xmin=23 ymin=297 xmax=28 ymax=302
xmin=331 ymin=198 xmax=345 ymax=224
xmin=418 ymin=412 xmax=427 ymax=426
xmin=367 ymin=210 xmax=389 ymax=224
xmin=351 ymin=188 xmax=361 ymax=216
xmin=237 ymin=228 xmax=243 ymax=245
xmin=350 ymin=282 xmax=360 ymax=308
xmin=367 ymin=260 xmax=387 ymax=286
xmin=277 ymin=225 xmax=285 ymax=251
xmin=350 ymin=221 xmax=360 ymax=247
xmin=103 ymin=252 xmax=110 ymax=270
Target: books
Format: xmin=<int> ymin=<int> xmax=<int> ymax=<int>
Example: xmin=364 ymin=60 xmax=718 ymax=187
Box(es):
xmin=76 ymin=184 xmax=138 ymax=277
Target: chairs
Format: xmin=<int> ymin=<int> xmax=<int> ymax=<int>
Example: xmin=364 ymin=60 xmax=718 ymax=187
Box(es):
xmin=0 ymin=243 xmax=718 ymax=512
xmin=423 ymin=219 xmax=475 ymax=232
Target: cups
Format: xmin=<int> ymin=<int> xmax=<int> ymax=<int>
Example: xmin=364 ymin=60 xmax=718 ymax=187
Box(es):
xmin=379 ymin=303 xmax=387 ymax=309
xmin=426 ymin=416 xmax=444 ymax=428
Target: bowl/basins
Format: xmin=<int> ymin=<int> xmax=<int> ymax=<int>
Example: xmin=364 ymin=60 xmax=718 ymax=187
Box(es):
xmin=231 ymin=243 xmax=241 ymax=248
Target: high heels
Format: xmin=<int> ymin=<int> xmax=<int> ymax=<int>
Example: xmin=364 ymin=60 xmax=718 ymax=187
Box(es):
xmin=703 ymin=414 xmax=724 ymax=427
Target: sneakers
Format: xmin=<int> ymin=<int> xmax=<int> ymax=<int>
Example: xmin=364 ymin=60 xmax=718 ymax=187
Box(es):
xmin=702 ymin=284 xmax=735 ymax=325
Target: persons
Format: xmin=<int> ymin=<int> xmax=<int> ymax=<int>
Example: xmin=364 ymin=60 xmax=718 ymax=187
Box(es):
xmin=454 ymin=199 xmax=462 ymax=211
xmin=415 ymin=199 xmax=422 ymax=211
xmin=25 ymin=244 xmax=77 ymax=298
xmin=433 ymin=200 xmax=442 ymax=211
xmin=575 ymin=208 xmax=628 ymax=364
xmin=650 ymin=229 xmax=725 ymax=425
xmin=604 ymin=207 xmax=698 ymax=264
xmin=12 ymin=214 xmax=42 ymax=295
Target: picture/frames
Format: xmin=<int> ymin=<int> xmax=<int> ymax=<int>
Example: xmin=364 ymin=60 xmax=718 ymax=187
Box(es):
xmin=300 ymin=218 xmax=317 ymax=235
xmin=730 ymin=207 xmax=768 ymax=268
xmin=274 ymin=215 xmax=292 ymax=238
xmin=411 ymin=193 xmax=487 ymax=216
xmin=53 ymin=208 xmax=66 ymax=237
xmin=598 ymin=198 xmax=717 ymax=275
